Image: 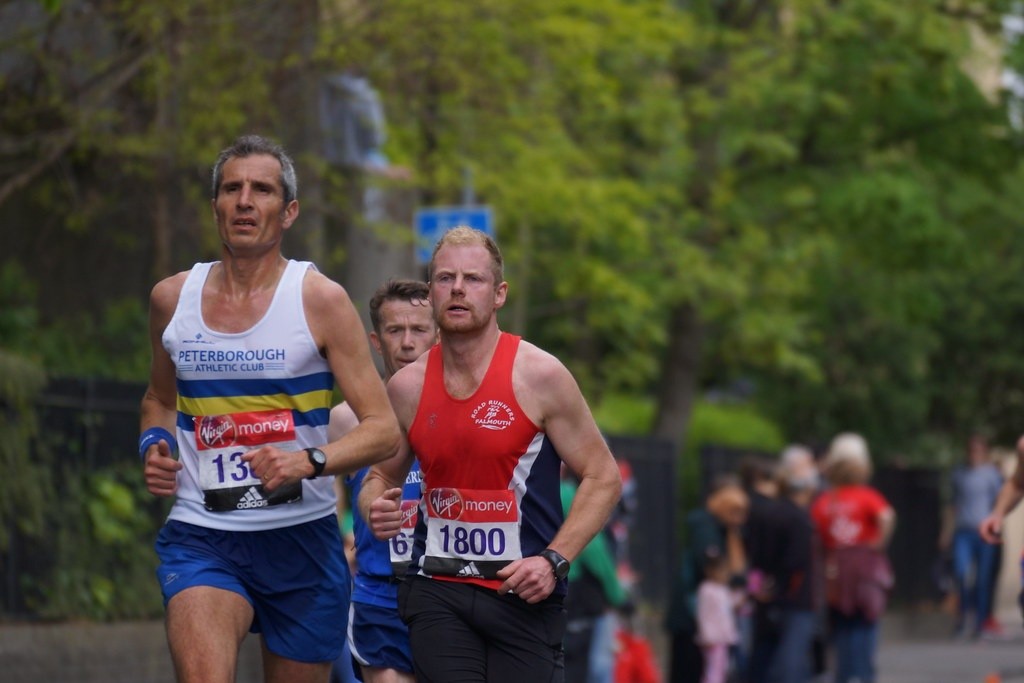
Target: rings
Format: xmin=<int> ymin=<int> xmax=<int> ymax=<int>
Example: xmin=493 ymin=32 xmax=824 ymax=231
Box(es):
xmin=263 ymin=474 xmax=270 ymax=481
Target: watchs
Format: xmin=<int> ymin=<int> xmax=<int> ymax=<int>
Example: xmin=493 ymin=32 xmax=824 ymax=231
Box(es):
xmin=538 ymin=549 xmax=571 ymax=581
xmin=303 ymin=447 xmax=325 ymax=480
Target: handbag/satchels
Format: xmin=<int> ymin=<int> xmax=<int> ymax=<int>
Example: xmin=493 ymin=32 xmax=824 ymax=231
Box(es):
xmin=567 ymin=565 xmax=604 ymax=620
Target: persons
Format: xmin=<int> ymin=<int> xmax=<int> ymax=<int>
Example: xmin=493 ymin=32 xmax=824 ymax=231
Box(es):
xmin=137 ymin=134 xmax=402 ymax=683
xmin=980 ymin=435 xmax=1024 ymax=622
xmin=666 ymin=434 xmax=897 ymax=683
xmin=326 ymin=279 xmax=439 ymax=683
xmin=559 ymin=462 xmax=662 ymax=683
xmin=937 ymin=430 xmax=1003 ymax=640
xmin=357 ymin=228 xmax=622 ymax=683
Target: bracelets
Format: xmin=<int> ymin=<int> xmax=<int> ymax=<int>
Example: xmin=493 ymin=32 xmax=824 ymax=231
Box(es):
xmin=139 ymin=427 xmax=177 ymax=464
